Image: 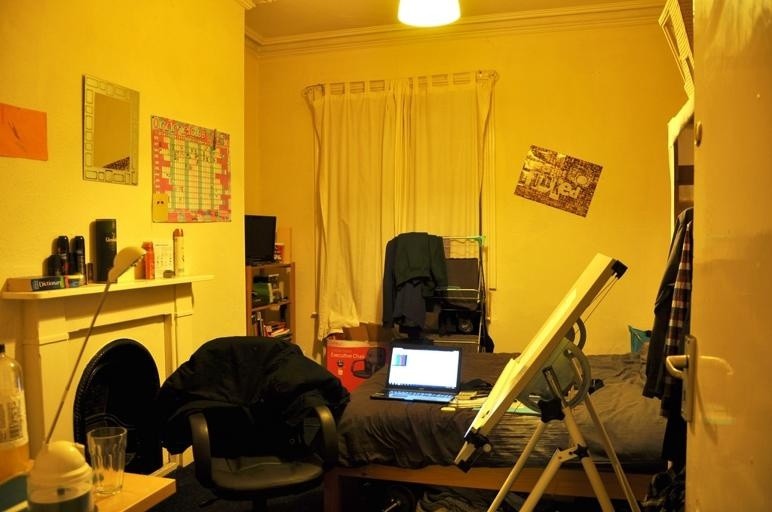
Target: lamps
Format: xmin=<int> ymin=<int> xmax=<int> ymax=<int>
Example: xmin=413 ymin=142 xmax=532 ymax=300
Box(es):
xmin=38 ymin=248 xmax=147 ymax=443
xmin=398 ymin=0 xmax=461 ymax=26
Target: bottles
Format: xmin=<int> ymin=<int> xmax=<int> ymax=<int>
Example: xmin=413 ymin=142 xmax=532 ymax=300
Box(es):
xmin=73 ymin=235 xmax=87 ymax=286
xmin=142 ymin=241 xmax=156 ymax=280
xmin=0 ymin=344 xmax=29 ymax=479
xmin=96 ymin=218 xmax=117 ymax=283
xmin=57 ymin=234 xmax=70 ymax=286
xmin=174 ymin=228 xmax=184 ymax=276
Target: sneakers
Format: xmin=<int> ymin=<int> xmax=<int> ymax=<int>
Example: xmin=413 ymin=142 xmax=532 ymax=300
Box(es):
xmin=414 ymin=487 xmax=545 ymax=512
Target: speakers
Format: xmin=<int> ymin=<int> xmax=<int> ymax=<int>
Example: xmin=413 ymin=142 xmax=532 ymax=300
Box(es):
xmin=439 ymin=309 xmax=481 ymax=335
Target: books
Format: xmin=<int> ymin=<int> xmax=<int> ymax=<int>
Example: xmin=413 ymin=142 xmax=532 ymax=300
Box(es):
xmin=449 ymin=389 xmax=542 ymax=416
xmin=251 ymin=311 xmax=292 ymax=340
xmin=253 ymin=281 xmax=274 ymax=307
xmin=6 ymin=275 xmax=62 ymax=292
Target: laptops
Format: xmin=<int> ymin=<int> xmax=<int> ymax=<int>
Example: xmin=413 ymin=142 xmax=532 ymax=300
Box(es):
xmin=370 ymin=344 xmax=463 ymax=404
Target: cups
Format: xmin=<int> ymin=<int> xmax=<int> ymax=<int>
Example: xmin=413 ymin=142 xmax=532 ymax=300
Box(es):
xmin=86 ymin=425 xmax=129 ymax=497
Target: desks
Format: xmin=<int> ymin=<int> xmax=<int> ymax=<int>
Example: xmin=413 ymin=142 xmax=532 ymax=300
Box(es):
xmin=0 ymin=459 xmax=177 ymax=512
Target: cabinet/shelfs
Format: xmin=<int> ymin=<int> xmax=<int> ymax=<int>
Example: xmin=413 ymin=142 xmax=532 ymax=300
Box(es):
xmin=407 ymin=236 xmax=486 ymax=352
xmin=246 ymin=262 xmax=296 ymax=344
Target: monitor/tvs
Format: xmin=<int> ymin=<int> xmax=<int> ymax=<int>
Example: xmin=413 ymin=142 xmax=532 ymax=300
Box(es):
xmin=245 ymin=215 xmax=277 ymax=266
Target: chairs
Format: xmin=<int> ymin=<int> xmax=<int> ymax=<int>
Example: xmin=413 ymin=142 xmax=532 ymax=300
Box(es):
xmin=188 ymin=336 xmax=339 ymax=512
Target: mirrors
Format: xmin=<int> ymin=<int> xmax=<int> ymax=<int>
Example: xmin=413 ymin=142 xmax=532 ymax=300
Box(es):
xmin=83 ymin=73 xmax=139 ymax=185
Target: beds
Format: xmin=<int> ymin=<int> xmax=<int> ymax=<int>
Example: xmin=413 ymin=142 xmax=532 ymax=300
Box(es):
xmin=324 ymin=352 xmax=668 ymax=512
